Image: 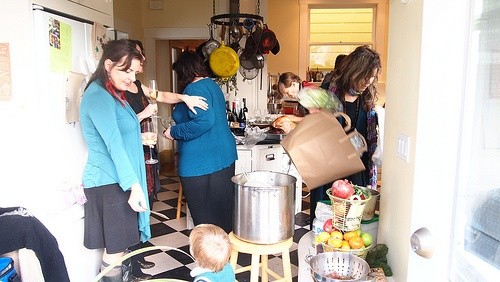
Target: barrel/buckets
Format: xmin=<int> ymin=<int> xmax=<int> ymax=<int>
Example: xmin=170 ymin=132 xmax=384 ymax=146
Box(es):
xmin=362 ymin=189 xmax=380 ymax=221
xmin=231 ymin=169 xmax=297 ymax=245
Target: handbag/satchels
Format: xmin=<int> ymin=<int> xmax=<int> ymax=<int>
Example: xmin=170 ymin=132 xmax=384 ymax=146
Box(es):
xmin=280 ymin=109 xmax=367 ymax=191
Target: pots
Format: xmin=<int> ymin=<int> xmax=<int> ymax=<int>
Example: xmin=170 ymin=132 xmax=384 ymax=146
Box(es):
xmin=201 ymin=20 xmax=280 ymax=81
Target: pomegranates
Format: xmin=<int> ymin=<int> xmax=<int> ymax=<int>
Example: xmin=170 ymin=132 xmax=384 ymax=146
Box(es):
xmin=323 ymin=219 xmax=340 ymax=234
xmin=331 ymin=179 xmax=354 ymax=199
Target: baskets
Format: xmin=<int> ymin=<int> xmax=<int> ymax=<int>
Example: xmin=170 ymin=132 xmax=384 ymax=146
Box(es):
xmin=326 ymin=188 xmax=372 ymax=232
xmin=322 ymin=243 xmax=373 ymax=261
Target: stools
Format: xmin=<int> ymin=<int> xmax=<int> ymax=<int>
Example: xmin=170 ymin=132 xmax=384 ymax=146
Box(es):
xmin=228 ymin=231 xmax=293 ymax=282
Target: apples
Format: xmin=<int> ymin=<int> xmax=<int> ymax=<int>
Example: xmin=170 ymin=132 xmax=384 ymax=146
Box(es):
xmin=343 ymin=229 xmax=373 ymax=250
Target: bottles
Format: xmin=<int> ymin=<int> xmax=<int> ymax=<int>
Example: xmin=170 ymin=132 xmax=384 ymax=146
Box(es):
xmin=225 ymin=97 xmax=249 ymax=127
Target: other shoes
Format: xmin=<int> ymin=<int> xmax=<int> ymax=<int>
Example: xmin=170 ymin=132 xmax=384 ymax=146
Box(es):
xmin=130 ymin=266 xmax=153 ymax=282
xmin=135 ymin=254 xmax=155 ymax=269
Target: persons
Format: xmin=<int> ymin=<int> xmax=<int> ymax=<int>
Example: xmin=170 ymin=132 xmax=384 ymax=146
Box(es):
xmin=80 ymin=39 xmax=158 ymax=282
xmin=163 ymin=49 xmax=238 ymax=233
xmin=189 ymin=224 xmax=237 ymax=282
xmin=279 ymin=44 xmax=384 ymax=229
xmin=124 ymin=40 xmax=209 ymax=281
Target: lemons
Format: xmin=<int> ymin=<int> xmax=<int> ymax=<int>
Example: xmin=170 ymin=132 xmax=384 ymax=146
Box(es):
xmin=318 ymin=231 xmax=350 ymax=250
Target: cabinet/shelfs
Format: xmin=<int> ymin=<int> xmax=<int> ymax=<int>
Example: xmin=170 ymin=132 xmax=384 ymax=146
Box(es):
xmin=186 ymin=145 xmax=302 ymax=231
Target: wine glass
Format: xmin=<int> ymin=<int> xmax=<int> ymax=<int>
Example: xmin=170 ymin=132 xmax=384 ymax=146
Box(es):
xmin=148 ymin=79 xmax=161 ymax=119
xmin=143 ymin=122 xmax=158 ymax=164
xmin=254 ymin=108 xmax=281 ymax=123
